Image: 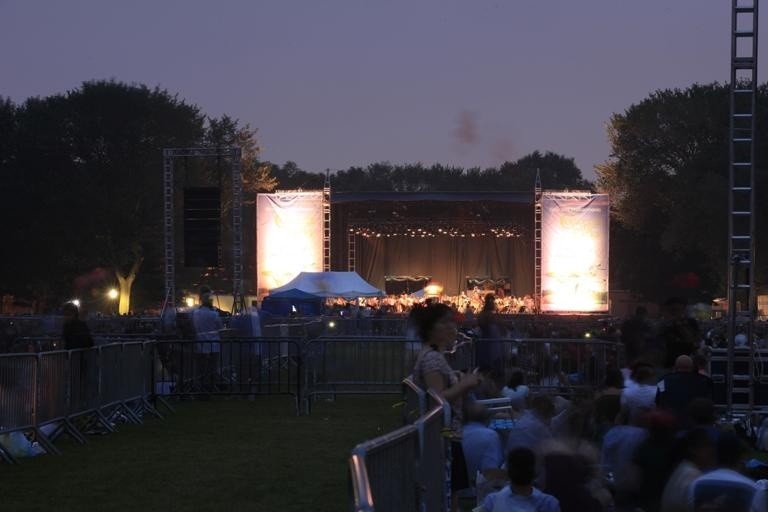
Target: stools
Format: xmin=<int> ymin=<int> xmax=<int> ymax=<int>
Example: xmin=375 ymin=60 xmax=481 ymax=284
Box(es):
xmin=477 ymin=397 xmax=521 ymax=431
